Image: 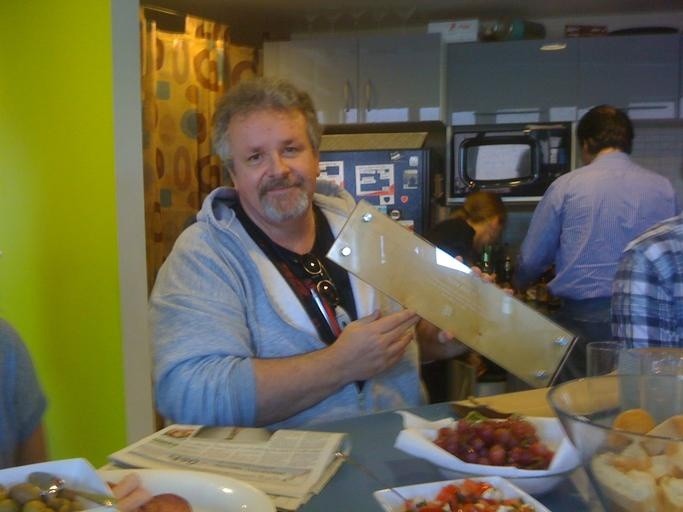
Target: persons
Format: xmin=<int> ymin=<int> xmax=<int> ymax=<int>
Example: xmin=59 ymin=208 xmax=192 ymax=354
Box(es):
xmin=608 ymin=212 xmax=683 ymax=357
xmin=149 ymin=75 xmax=513 ymax=432
xmin=0 ymin=318 xmax=46 ymax=470
xmin=417 ymin=191 xmax=508 ymax=404
xmin=515 ymin=105 xmax=682 ymax=387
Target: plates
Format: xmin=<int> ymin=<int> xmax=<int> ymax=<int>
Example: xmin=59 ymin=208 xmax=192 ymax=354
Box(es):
xmin=371 ymin=475 xmax=552 ymax=512
xmin=99 ymin=469 xmax=278 ymax=512
xmin=0 ymin=456 xmax=120 ymax=512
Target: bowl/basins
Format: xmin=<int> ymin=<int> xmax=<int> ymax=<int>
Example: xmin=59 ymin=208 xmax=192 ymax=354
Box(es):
xmin=393 ymin=409 xmax=606 ymax=495
xmin=546 ymin=373 xmax=683 ymax=511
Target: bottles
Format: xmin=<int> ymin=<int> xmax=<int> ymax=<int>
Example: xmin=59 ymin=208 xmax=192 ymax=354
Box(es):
xmin=510 ymin=276 xmax=558 ymax=312
xmin=481 ymin=244 xmax=494 ymax=274
xmin=502 ymin=256 xmax=513 ymax=289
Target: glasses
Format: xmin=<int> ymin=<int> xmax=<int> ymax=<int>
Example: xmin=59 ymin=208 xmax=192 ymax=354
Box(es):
xmin=300 ymin=253 xmax=341 ymax=307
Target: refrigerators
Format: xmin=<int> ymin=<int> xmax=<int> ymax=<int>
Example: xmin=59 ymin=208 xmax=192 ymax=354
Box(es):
xmin=314 ymin=131 xmax=443 ymax=236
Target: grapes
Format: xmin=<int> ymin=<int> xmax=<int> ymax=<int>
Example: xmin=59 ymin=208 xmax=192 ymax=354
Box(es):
xmin=436 ymin=412 xmax=554 ymax=469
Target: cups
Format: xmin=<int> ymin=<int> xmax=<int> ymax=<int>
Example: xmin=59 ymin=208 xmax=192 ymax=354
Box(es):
xmin=586 ymin=341 xmax=627 ymax=395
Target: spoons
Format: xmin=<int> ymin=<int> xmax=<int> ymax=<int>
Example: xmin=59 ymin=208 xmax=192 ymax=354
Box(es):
xmin=28 ymin=471 xmax=118 ymax=508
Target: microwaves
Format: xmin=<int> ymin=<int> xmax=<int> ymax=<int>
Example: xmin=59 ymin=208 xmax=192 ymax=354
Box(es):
xmin=451 ymin=124 xmax=572 ymax=197
